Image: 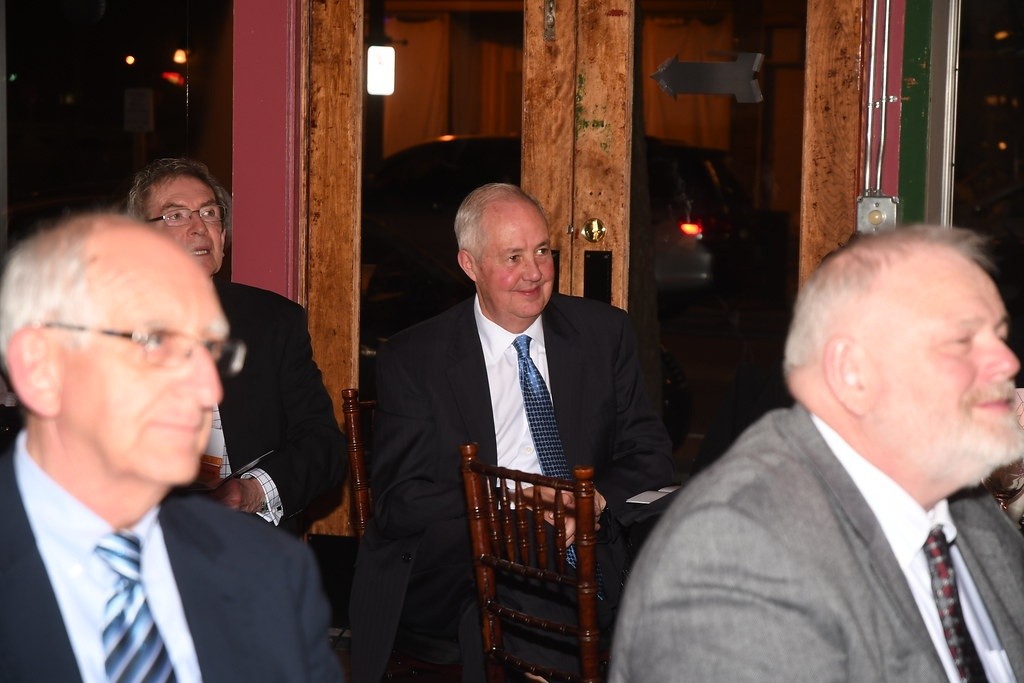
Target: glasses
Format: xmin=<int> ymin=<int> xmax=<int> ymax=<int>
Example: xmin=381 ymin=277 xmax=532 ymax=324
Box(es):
xmin=145 ymin=204 xmax=228 ymax=227
xmin=41 ymin=319 xmax=225 ymax=370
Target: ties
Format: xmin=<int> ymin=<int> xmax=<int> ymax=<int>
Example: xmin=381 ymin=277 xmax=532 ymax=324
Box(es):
xmin=924 ymin=526 xmax=992 ymax=682
xmin=512 ymin=335 xmax=571 ymax=482
xmin=93 ymin=530 xmax=178 ymax=682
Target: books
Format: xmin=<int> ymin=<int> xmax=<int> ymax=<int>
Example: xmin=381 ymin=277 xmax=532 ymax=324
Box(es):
xmin=625 ymin=485 xmax=680 ymax=504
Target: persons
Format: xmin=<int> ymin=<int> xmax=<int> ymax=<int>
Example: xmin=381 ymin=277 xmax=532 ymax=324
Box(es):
xmin=371 ymin=181 xmax=682 ymax=681
xmin=607 ymin=221 xmax=1022 ymax=679
xmin=2 ymin=211 xmax=344 ymax=682
xmin=126 ymin=157 xmax=351 ymax=532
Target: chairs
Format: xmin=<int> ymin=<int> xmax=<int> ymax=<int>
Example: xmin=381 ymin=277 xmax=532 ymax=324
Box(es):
xmin=342 ymin=389 xmax=463 ymax=668
xmin=461 ymin=440 xmax=610 ymax=683
